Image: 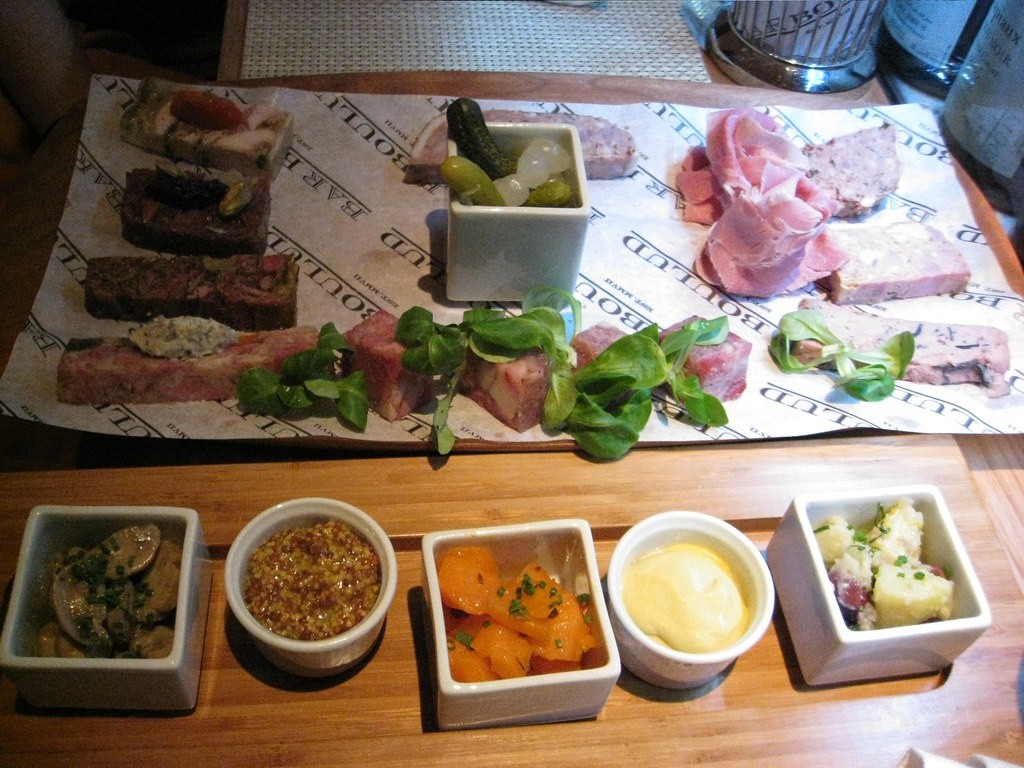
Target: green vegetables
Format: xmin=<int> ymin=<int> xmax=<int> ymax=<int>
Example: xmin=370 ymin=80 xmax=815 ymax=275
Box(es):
xmin=230 ymin=286 xmax=915 ymax=461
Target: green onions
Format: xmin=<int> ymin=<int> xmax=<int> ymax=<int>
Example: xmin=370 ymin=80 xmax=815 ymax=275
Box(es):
xmin=445 ymin=573 xmax=593 ymax=650
xmin=814 ymin=504 xmax=953 ymax=581
xmin=56 ymin=535 xmax=176 ymax=658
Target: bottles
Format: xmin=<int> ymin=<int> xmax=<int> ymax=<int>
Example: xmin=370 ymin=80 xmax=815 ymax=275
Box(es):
xmin=936 ymin=0 xmax=1024 ymax=217
xmin=874 ymin=0 xmax=993 ymax=101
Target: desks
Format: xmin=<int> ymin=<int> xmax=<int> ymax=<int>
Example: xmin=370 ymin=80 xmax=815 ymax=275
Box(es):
xmin=0 ymin=0 xmax=1024 ymax=768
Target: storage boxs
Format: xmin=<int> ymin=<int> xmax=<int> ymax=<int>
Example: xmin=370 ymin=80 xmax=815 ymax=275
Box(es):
xmin=769 ymin=483 xmax=992 ymax=686
xmin=417 ymin=517 xmax=622 ymax=731
xmin=0 ymin=504 xmax=212 ymax=712
xmin=441 ymin=122 xmax=593 ymax=302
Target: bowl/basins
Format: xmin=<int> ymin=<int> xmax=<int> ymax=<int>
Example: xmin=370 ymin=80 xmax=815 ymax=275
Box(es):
xmin=605 ymin=509 xmax=776 ymax=689
xmin=420 ymin=518 xmax=623 ymax=731
xmin=223 ymin=497 xmax=398 ymax=676
xmin=767 ymin=485 xmax=990 ymax=687
xmin=0 ymin=504 xmax=211 ymax=712
xmin=444 ymin=121 xmax=591 ymax=304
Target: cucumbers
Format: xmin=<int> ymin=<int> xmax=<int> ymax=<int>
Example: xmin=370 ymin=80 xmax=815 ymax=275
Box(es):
xmin=444 ymin=98 xmax=574 ymax=208
